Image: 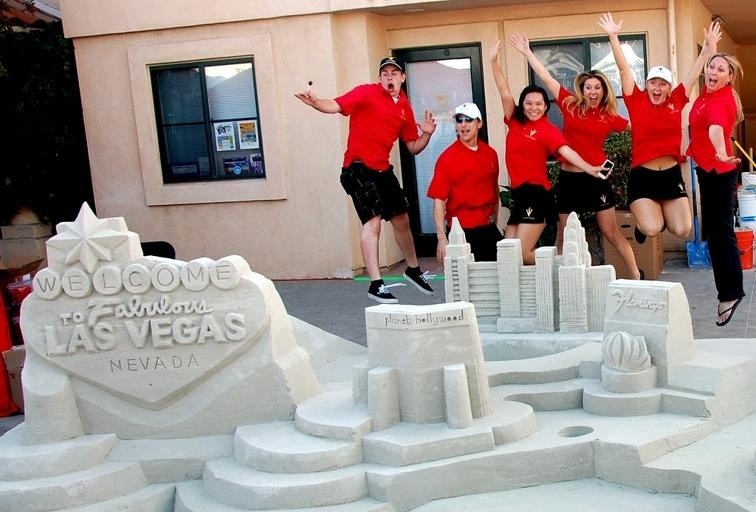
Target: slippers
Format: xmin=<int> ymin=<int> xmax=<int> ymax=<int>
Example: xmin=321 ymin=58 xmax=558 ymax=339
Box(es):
xmin=716 ymin=297 xmax=743 ymax=326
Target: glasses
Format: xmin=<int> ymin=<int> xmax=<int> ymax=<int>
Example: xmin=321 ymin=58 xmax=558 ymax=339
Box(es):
xmin=456 ymin=115 xmax=476 ymax=123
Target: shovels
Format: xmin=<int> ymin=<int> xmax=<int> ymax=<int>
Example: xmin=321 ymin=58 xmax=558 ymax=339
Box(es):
xmin=685 ymin=125 xmax=712 ymax=268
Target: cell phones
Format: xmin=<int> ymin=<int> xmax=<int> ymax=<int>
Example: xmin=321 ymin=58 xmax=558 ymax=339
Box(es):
xmin=597 ymin=159 xmax=614 ymax=180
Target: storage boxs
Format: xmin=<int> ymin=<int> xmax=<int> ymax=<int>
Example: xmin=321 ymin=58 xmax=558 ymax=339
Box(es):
xmin=604 ymin=209 xmax=664 ymax=280
xmin=1 ymin=345 xmax=27 ymax=414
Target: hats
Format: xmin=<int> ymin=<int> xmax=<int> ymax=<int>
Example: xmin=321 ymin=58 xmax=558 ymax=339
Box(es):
xmin=380 ymin=56 xmax=405 ymax=73
xmin=452 ymin=103 xmax=482 ymax=120
xmin=647 ymin=65 xmax=673 ymax=83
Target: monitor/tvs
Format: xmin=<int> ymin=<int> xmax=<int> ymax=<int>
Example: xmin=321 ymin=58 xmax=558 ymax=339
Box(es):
xmin=170 ymin=161 xmax=200 ymax=176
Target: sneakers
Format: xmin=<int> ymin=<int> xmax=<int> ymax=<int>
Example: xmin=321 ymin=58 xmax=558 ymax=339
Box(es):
xmin=367 ymin=279 xmax=399 ymax=303
xmin=639 ymin=269 xmax=644 ymax=280
xmin=403 ymin=265 xmax=436 ymax=296
xmin=635 ymin=224 xmax=645 ymax=244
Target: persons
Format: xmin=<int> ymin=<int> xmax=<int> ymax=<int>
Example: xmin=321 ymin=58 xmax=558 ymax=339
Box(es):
xmin=507 ymin=29 xmax=645 ymax=281
xmin=293 ymin=55 xmax=437 ymax=303
xmin=685 ymin=33 xmax=746 ymax=327
xmin=426 ymin=99 xmax=504 ymax=265
xmin=490 ymin=38 xmax=614 ymax=265
xmin=594 ymin=10 xmax=723 ymax=245
